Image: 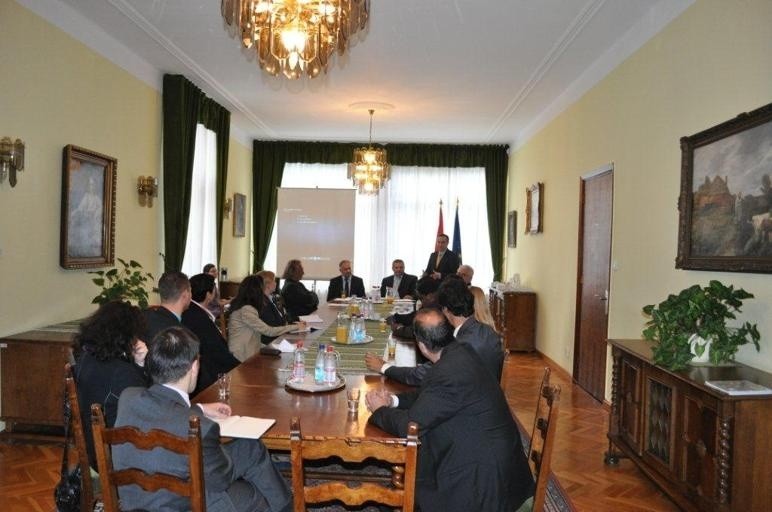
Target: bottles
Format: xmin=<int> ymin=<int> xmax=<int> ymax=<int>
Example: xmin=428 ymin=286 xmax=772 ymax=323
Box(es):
xmin=293 ymin=342 xmax=305 ymax=384
xmin=314 ymin=343 xmax=326 ymax=385
xmin=371 ymin=286 xmax=381 ymax=303
xmin=324 ymin=346 xmax=337 ymax=385
xmin=360 ymin=313 xmax=366 ymax=338
xmin=349 ymin=313 xmax=356 ymax=340
xmin=355 ymin=315 xmax=363 ymax=342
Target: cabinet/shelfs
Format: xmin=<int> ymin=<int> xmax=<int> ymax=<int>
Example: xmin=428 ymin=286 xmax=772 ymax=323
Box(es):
xmin=605 ymin=339 xmax=772 ymax=512
xmin=0 ymin=317 xmax=88 ymax=445
xmin=489 ymin=286 xmax=536 ymax=355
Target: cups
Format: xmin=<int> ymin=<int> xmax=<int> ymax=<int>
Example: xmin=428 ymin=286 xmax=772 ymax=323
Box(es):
xmin=384 ymin=287 xmax=400 ymax=306
xmin=218 ymin=373 xmax=231 ymax=396
xmin=388 ymin=339 xmax=397 ymax=357
xmin=336 ymin=324 xmax=347 ymax=344
xmin=347 ymin=388 xmax=360 ymax=412
xmin=338 ymin=290 xmax=369 ymax=314
xmin=379 ymin=320 xmax=387 ymax=332
xmin=366 ymin=351 xmax=377 ymax=369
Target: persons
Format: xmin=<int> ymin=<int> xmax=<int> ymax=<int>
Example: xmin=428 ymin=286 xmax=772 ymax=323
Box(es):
xmin=74 ymin=300 xmax=151 ymax=473
xmin=142 ymin=259 xmax=320 ymax=399
xmin=70 ymin=176 xmax=103 ymax=257
xmin=327 ymin=260 xmax=366 ymax=303
xmin=424 ymin=234 xmax=459 ymax=281
xmin=111 ymin=325 xmax=294 ymax=511
xmin=366 ymin=258 xmax=507 ymax=385
xmin=365 ymin=306 xmax=537 ymax=512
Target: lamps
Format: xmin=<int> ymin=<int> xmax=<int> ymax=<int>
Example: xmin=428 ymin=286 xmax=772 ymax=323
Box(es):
xmin=347 ymin=100 xmax=391 ymax=196
xmin=1 ymin=134 xmax=25 ymax=186
xmin=221 ymin=0 xmax=369 ymax=91
xmin=137 ymin=175 xmax=159 ymax=209
xmin=224 ymin=199 xmax=232 ymax=221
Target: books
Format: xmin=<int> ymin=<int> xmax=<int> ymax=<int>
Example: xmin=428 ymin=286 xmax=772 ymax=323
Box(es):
xmin=290 ymin=325 xmax=322 ymax=334
xmin=704 ymin=380 xmax=772 ymax=396
xmin=210 ymin=414 xmax=276 ymax=440
xmin=298 ymin=315 xmax=323 ymax=323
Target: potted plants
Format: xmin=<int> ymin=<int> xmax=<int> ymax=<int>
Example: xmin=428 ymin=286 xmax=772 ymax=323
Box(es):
xmin=641 ymin=280 xmax=762 ymax=372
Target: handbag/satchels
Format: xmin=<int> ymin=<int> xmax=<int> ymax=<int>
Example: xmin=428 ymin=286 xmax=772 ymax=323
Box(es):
xmin=207 ymin=302 xmax=222 ymax=317
xmin=54 ymin=463 xmax=84 ymax=512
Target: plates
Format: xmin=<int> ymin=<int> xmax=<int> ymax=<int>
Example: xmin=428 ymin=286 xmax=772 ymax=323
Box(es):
xmin=286 ymin=369 xmax=346 ymax=392
xmin=331 ymin=333 xmax=374 ymax=344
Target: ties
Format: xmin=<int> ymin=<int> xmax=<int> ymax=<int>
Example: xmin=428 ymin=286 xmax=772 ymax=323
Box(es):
xmin=436 ymin=255 xmax=442 ymax=266
xmin=345 ymin=278 xmax=350 ymax=296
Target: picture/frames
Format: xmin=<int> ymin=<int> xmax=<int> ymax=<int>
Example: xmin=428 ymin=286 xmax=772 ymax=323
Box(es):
xmin=525 ymin=181 xmax=543 ymax=233
xmin=59 ymin=143 xmax=117 ymax=271
xmin=674 ymin=103 xmax=772 ymax=275
xmin=233 ymin=193 xmax=246 ymax=236
xmin=507 ymin=211 xmax=516 ymax=249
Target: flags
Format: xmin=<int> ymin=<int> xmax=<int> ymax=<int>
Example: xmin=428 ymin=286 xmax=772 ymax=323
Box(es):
xmin=452 ymin=206 xmax=462 ymax=265
xmin=434 ymin=209 xmax=443 ymax=252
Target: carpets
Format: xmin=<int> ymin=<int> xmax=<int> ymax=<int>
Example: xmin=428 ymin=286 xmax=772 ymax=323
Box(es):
xmin=92 ymin=404 xmax=578 ymax=512
